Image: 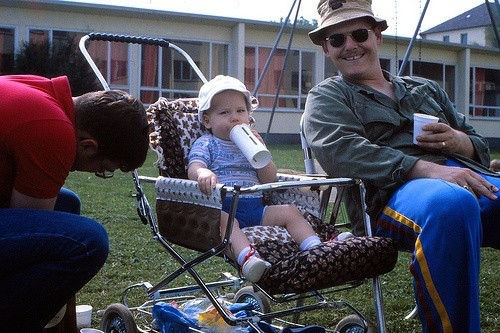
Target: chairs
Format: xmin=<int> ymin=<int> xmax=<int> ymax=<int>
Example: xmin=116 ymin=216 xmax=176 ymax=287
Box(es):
xmin=299 ymin=112 xmax=418 ymax=320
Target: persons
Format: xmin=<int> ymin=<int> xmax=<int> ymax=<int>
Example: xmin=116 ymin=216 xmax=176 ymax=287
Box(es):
xmin=0 ymin=75 xmax=150 ymax=333
xmin=188 ymin=75 xmax=355 ymax=282
xmin=300 ymin=0 xmax=500 ymax=333
xmin=489 ymin=159 xmax=500 ymax=172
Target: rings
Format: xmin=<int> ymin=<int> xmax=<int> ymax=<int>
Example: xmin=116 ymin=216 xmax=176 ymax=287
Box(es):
xmin=463 ymin=185 xmax=468 ymax=190
xmin=442 ymin=141 xmax=446 ymax=147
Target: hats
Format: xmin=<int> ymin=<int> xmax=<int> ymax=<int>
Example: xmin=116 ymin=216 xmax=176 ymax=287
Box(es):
xmin=308 ymin=0 xmax=388 ymax=45
xmin=197 ymin=74 xmax=252 ymax=122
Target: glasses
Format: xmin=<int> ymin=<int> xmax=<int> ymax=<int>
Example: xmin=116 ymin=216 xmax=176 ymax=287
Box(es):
xmin=324 ymin=28 xmax=373 ymax=48
xmin=80 ymin=137 xmax=115 ymax=179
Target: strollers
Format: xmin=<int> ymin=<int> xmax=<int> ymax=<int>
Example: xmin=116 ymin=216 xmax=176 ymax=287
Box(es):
xmin=78 ymin=32 xmax=398 ymax=333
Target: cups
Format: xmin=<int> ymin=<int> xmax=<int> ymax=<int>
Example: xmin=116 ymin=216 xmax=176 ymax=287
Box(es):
xmin=229 ymin=123 xmax=272 ymax=169
xmin=413 ymin=113 xmax=439 ymax=145
xmin=75 ymin=305 xmax=92 ymax=329
xmin=79 ymin=328 xmax=104 ymax=333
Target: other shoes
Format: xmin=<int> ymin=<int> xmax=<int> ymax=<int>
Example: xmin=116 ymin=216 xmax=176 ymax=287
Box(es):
xmin=240 ymin=244 xmax=272 ymax=281
xmin=332 ymin=232 xmax=353 ymax=240
